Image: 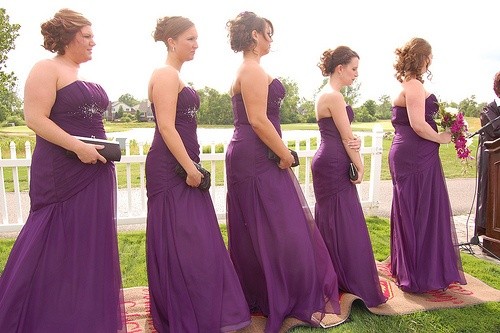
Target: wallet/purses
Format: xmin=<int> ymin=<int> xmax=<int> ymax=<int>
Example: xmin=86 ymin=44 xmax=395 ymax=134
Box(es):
xmin=178 ymin=158 xmax=211 ymax=190
xmin=268 ymin=143 xmax=300 ymax=167
xmin=68 ymin=134 xmax=121 ymax=161
xmin=349 ymin=162 xmax=359 ymax=181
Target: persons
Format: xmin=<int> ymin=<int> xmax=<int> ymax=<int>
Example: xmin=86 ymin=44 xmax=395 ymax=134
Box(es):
xmin=388 ymin=38 xmax=467 ymax=294
xmin=474 ymin=72 xmax=500 ymax=242
xmin=225 ymin=12 xmax=341 ymax=333
xmin=311 ymin=46 xmax=387 ymax=308
xmin=0 ymin=9 xmax=128 ymax=333
xmin=145 ymin=17 xmax=251 ymax=333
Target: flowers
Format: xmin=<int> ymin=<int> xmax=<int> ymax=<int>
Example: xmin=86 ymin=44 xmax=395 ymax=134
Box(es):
xmin=432 ymin=102 xmax=476 ymax=176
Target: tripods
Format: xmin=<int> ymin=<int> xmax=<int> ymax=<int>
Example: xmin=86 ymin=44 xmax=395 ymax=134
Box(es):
xmin=454 ymin=116 xmax=500 ymax=261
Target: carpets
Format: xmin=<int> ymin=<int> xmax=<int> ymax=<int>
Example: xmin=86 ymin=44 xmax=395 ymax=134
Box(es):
xmin=119 ymin=255 xmax=500 ymax=333
